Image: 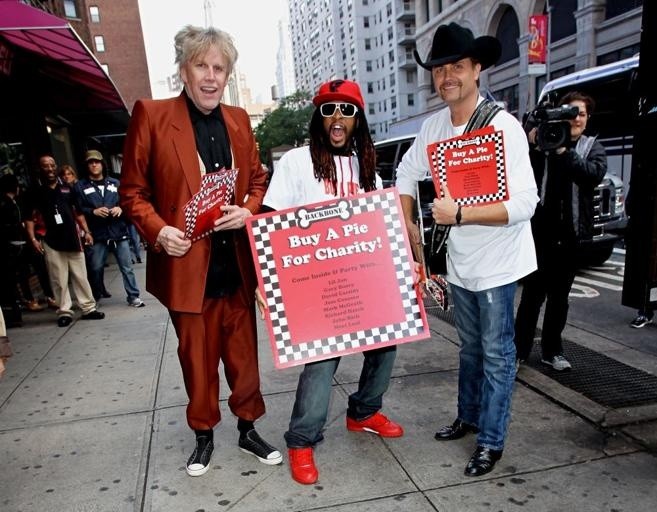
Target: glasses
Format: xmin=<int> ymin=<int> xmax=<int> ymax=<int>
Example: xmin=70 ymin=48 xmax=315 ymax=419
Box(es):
xmin=318 ymin=102 xmax=358 ymax=117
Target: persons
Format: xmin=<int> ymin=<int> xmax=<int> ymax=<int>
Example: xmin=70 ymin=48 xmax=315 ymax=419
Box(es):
xmin=2 ymin=144 xmax=148 ymax=327
xmin=117 ymin=22 xmax=283 ymax=476
xmin=248 ymin=79 xmax=404 ymax=483
xmin=0 ymin=308 xmax=11 ymax=389
xmin=393 ymin=22 xmax=541 ymax=477
xmin=515 ymin=90 xmax=609 ymax=370
xmin=621 ymin=82 xmax=657 ymax=330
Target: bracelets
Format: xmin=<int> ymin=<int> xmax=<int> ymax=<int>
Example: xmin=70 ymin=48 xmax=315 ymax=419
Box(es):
xmin=455 ymin=205 xmax=461 ymax=226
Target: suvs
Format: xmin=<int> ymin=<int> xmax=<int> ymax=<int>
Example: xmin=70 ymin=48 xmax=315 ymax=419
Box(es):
xmin=368 ymin=134 xmax=628 ymax=279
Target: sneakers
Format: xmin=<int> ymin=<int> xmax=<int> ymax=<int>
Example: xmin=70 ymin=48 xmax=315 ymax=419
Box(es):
xmin=239 ymin=427 xmax=282 ymax=465
xmin=289 ymin=446 xmax=318 ymax=484
xmin=629 ymin=315 xmax=653 ymax=328
xmin=346 ymin=412 xmax=404 ymax=437
xmin=129 ymin=296 xmax=145 ymax=307
xmin=541 ymin=354 xmax=571 ymax=369
xmin=186 ymin=435 xmax=214 ymax=477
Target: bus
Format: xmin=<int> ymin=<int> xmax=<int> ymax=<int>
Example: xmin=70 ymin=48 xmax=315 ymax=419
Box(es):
xmin=532 ymin=55 xmax=640 ymax=227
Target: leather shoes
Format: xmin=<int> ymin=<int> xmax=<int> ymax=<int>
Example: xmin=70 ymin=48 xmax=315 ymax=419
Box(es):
xmin=57 ymin=317 xmax=71 ymax=327
xmin=48 ymin=298 xmax=59 ymax=307
xmin=465 ymin=446 xmax=502 ymax=476
xmin=28 ymin=301 xmax=44 ymax=311
xmin=435 ymin=418 xmax=481 ymax=440
xmin=83 ymin=311 xmax=104 ymax=319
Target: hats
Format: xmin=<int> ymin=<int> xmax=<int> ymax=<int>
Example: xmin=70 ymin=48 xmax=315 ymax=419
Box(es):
xmin=313 ymin=80 xmax=364 ymax=110
xmin=414 ymin=23 xmax=501 ymax=72
xmin=86 ymin=150 xmax=103 ymax=161
